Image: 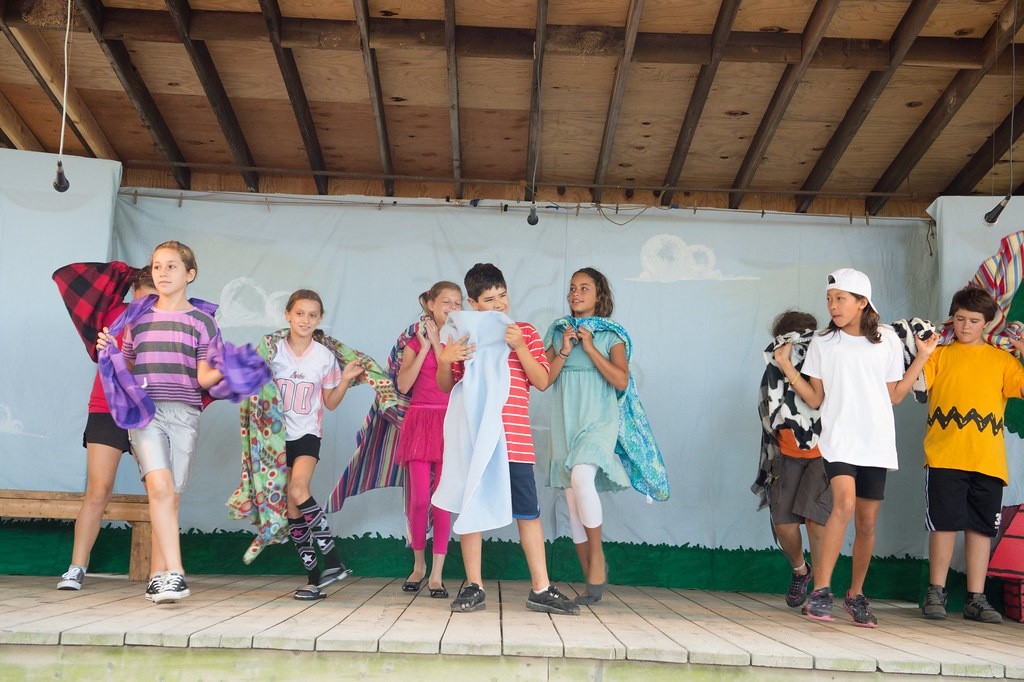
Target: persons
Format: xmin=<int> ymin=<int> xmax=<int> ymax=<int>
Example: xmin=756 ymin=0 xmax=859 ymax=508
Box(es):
xmin=227 ymin=289 xmax=365 ymax=600
xmin=922 ymin=287 xmax=1024 ymax=623
xmin=436 ymin=263 xmax=581 ymax=615
xmin=542 ymin=267 xmax=630 ymax=605
xmin=96 ymin=240 xmax=273 ymax=603
xmin=750 ymin=311 xmax=834 ymax=608
xmin=774 ymin=269 xmax=941 ymax=628
xmin=52 ymin=260 xmax=147 ymax=590
xmin=396 ymin=281 xmax=449 ymax=599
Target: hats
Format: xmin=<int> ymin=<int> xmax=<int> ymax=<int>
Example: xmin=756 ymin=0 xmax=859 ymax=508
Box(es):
xmin=825 ymin=269 xmax=882 ymax=315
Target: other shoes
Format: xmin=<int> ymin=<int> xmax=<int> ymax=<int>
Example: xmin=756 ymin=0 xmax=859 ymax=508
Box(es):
xmin=425 ymin=581 xmax=449 ymax=600
xmin=401 ymin=569 xmax=428 ymax=592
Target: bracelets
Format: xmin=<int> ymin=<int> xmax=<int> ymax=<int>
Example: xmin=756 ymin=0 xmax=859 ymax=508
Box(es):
xmin=558 ymin=349 xmax=569 ymax=359
xmin=790 ymin=372 xmax=800 ymax=386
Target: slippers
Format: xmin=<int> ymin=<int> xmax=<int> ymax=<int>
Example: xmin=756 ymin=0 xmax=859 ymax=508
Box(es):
xmin=293 ymin=584 xmax=327 ymax=601
xmin=316 ymin=564 xmax=354 ymax=589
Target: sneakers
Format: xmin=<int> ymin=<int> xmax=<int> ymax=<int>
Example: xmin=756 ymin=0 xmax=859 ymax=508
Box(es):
xmin=526 ymin=585 xmax=583 ymax=616
xmin=785 ymin=562 xmax=813 ymax=607
xmin=153 ymin=572 xmax=192 ymax=603
xmin=962 ymin=592 xmax=1003 ymax=625
xmin=804 ymin=587 xmax=836 ymax=622
xmin=452 ymin=582 xmax=487 ymax=612
xmin=55 ymin=565 xmax=86 ymax=591
xmin=920 ymin=584 xmax=949 ymax=621
xmin=841 ymin=589 xmax=879 ymax=628
xmin=145 ymin=577 xmax=168 ymax=599
